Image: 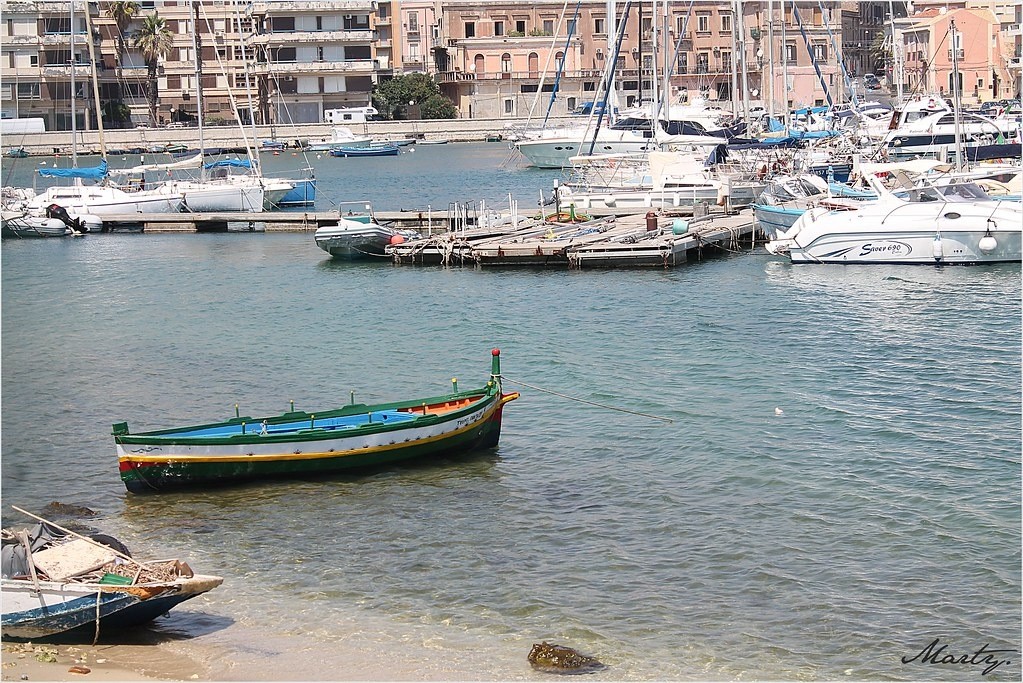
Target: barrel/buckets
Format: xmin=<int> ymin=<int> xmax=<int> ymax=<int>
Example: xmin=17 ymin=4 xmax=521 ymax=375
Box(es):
xmin=672 ymin=219 xmax=689 ymax=235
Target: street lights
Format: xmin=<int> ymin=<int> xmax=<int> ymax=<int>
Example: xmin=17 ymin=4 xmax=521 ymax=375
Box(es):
xmin=171 ymin=108 xmax=175 ymax=123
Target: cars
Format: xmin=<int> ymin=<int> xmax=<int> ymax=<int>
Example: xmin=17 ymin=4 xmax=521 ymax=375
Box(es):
xmin=365 ymin=114 xmax=392 ymax=121
xmin=862 ymin=73 xmax=881 ymax=91
xmin=981 ymin=99 xmax=1021 ymax=109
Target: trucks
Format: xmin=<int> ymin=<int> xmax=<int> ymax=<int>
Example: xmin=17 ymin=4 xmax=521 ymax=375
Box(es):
xmin=576 ymin=102 xmax=606 ymax=112
xmin=571 ymin=106 xmax=619 ymax=115
xmin=325 ymin=106 xmax=378 ymax=123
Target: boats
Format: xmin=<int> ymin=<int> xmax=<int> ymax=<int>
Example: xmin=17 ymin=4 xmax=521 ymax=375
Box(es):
xmin=485 ymin=136 xmax=500 ymax=143
xmin=417 ymin=139 xmax=448 ymax=145
xmin=108 ymin=148 xmax=123 ymax=156
xmin=263 ymin=140 xmax=284 ymax=149
xmin=111 ymin=349 xmax=521 ymax=496
xmin=8 ymin=148 xmax=29 ymax=159
xmin=166 ymin=144 xmax=188 ymax=153
xmin=10 ymin=214 xmax=103 ymax=237
xmin=308 ymin=128 xmax=373 ymax=151
xmin=2 ymin=559 xmax=224 ymax=645
xmin=331 ymin=137 xmax=417 ymax=157
xmin=148 ymin=147 xmax=165 ymax=152
xmin=314 ymin=200 xmax=421 ymax=258
xmin=753 ymin=115 xmax=1022 ymax=267
xmin=261 ymin=149 xmax=283 ymax=154
xmin=128 ymin=147 xmax=145 ymax=155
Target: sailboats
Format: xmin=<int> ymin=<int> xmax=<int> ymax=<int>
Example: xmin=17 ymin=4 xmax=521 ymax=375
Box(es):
xmin=513 ymin=2 xmax=933 ymax=211
xmin=1 ymin=0 xmax=317 ymax=213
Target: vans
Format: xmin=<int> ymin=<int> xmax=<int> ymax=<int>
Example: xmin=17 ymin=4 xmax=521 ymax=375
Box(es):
xmin=168 ymin=125 xmax=184 ymax=128
